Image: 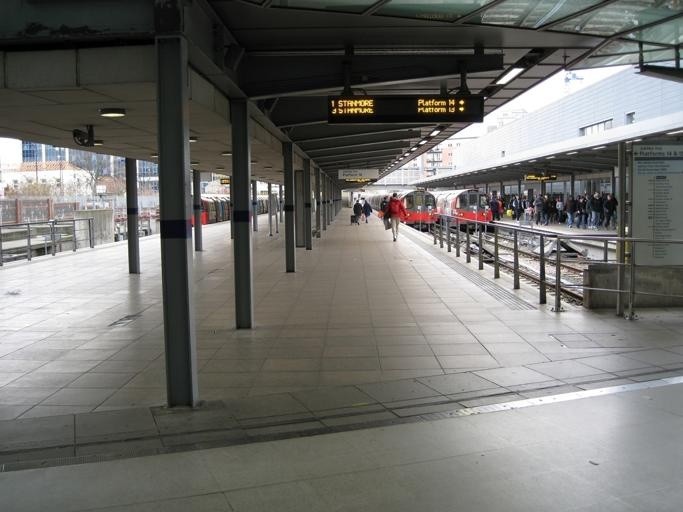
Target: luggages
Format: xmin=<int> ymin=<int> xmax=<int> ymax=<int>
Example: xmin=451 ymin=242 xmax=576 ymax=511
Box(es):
xmin=351 ymin=215 xmax=358 ymax=223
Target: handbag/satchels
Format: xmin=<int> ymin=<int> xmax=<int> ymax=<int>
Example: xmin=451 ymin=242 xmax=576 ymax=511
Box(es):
xmin=362 ymin=213 xmax=366 ymax=221
xmin=384 ymin=218 xmax=391 ymax=230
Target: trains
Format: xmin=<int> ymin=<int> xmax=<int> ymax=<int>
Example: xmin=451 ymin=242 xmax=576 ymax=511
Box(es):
xmin=353 ymin=186 xmax=495 ymax=235
xmin=191 ymin=192 xmax=285 ymax=227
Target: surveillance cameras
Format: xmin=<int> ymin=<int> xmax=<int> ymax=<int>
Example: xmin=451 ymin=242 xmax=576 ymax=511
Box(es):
xmin=73 ymin=129 xmax=88 ymax=145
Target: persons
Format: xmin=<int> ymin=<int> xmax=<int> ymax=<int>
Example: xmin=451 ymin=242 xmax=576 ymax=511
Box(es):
xmin=380 ymin=196 xmax=389 ymax=229
xmin=353 ymin=200 xmax=362 ymax=225
xmin=489 ymin=192 xmax=617 ymax=232
xmin=362 ymin=201 xmax=372 ymax=223
xmin=383 ymin=192 xmax=408 ymax=241
